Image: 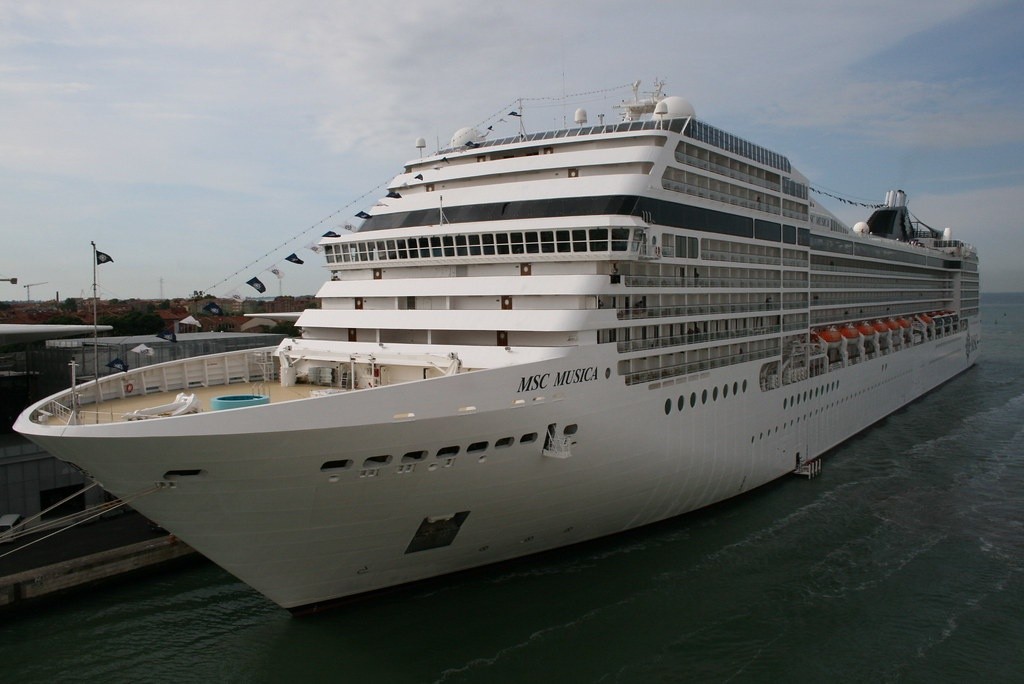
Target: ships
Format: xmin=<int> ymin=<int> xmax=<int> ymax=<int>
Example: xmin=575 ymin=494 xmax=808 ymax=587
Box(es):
xmin=12 ymin=76 xmax=981 ymax=620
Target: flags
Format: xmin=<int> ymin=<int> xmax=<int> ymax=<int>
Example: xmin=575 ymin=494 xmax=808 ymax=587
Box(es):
xmin=355 ymin=211 xmax=373 ymax=220
xmin=131 ymin=344 xmax=154 ymax=356
xmin=284 ymin=252 xmax=304 ymax=265
xmin=808 ymin=185 xmax=884 ymax=210
xmin=156 ymin=327 xmax=178 ymax=345
xmin=321 ymin=231 xmax=341 ymax=238
xmin=95 ymin=250 xmax=114 ymax=265
xmin=371 ymin=105 xmax=534 ymax=208
xmin=104 ymin=357 xmax=130 ymax=373
xmin=266 ymin=264 xmax=284 ymax=279
xmin=339 ymin=219 xmax=357 ymax=233
xmin=180 ymin=315 xmax=201 ymax=328
xmin=225 ymin=284 xmax=247 ymax=303
xmin=303 ymin=240 xmax=324 ymax=254
xmin=203 ymin=301 xmax=225 ymax=318
xmin=247 ymin=276 xmax=266 ymax=293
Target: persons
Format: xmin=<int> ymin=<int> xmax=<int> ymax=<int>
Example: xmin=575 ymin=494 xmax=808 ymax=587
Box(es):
xmin=629 ymin=265 xmax=925 ymax=360
